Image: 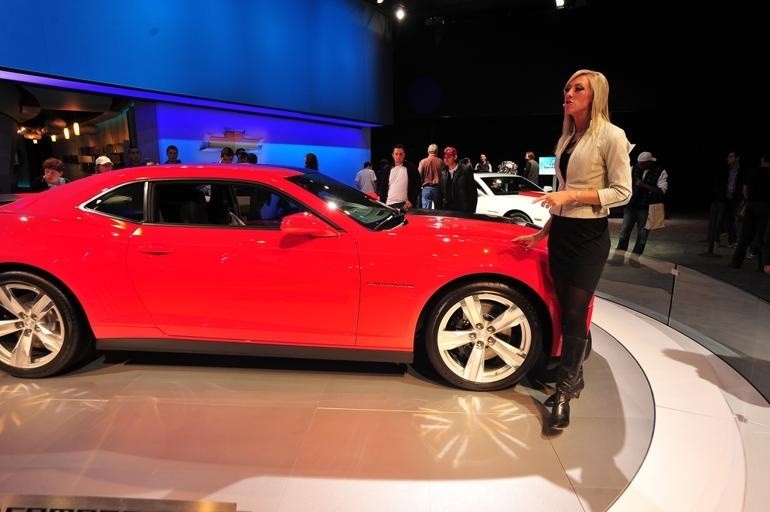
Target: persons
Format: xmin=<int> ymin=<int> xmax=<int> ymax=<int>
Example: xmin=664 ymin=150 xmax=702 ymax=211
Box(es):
xmin=534 ymin=68 xmax=635 ymax=431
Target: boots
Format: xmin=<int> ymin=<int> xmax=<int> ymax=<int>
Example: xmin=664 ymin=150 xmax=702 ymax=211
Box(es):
xmin=542 ymin=333 xmax=589 ymax=431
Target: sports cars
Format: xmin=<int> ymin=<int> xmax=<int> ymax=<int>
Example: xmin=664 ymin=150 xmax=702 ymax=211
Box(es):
xmin=1 ymin=160 xmax=596 ymax=391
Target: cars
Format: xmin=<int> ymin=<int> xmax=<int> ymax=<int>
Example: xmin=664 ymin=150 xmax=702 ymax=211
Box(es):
xmin=468 ymin=170 xmax=555 ymax=229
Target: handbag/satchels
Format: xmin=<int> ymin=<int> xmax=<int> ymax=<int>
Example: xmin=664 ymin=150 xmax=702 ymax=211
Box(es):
xmin=643 ymin=202 xmax=666 ymax=232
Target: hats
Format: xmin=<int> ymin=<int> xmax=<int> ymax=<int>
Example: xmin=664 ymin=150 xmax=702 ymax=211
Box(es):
xmin=443 ymin=146 xmax=457 ymax=155
xmin=427 ymin=144 xmax=438 ymax=152
xmin=637 ymin=151 xmax=656 ymax=163
xmin=95 ymin=155 xmax=113 ymax=166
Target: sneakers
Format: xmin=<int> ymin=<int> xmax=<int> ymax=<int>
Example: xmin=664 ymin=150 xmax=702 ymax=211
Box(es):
xmin=607 ymin=254 xmax=625 ymax=265
xmin=629 ymin=256 xmax=640 ymax=268
